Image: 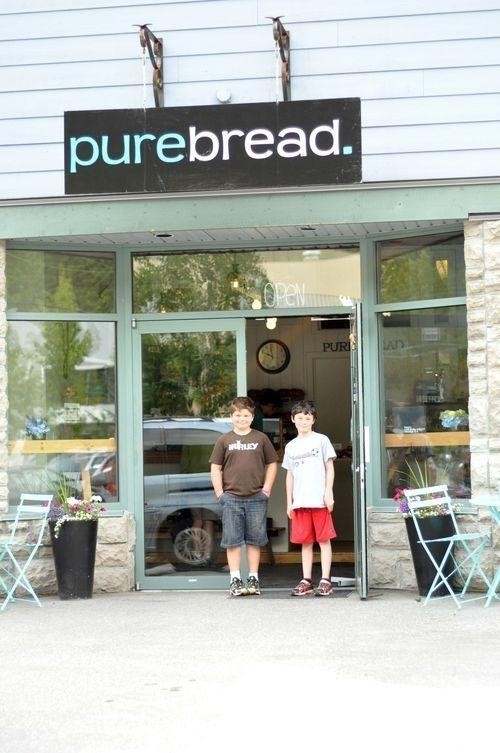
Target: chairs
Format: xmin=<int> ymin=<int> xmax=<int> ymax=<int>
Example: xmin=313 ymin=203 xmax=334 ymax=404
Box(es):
xmin=404 ymin=485 xmax=500 ymax=608
xmin=0 ymin=492 xmax=55 ymax=611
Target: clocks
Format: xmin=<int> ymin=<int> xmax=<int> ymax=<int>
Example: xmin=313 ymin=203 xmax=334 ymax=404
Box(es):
xmin=256 ymin=339 xmax=290 ymax=373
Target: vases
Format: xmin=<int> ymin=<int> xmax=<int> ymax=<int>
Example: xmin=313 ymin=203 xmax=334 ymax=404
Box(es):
xmin=48 ymin=521 xmax=97 ymax=600
xmin=405 ymin=516 xmax=456 ymax=596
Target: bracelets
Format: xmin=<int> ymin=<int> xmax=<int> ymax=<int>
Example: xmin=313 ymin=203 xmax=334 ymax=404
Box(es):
xmin=262 ymin=488 xmax=271 ymax=498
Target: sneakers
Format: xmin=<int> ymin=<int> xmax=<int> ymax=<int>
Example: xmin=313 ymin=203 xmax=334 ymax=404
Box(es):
xmin=313 ymin=578 xmax=333 ymax=596
xmin=291 ymin=578 xmax=314 ymax=596
xmin=229 ymin=578 xmax=249 ymax=597
xmin=191 ymin=555 xmax=213 ymax=571
xmin=246 ymin=576 xmax=261 ymax=594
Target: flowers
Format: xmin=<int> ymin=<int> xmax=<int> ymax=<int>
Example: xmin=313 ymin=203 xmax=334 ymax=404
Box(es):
xmin=45 ymin=469 xmax=105 ymax=539
xmin=390 ymin=460 xmax=457 ymax=516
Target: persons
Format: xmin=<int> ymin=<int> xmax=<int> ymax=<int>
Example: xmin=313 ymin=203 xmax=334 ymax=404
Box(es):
xmin=281 ymin=400 xmax=337 ymax=597
xmin=179 ymin=444 xmax=218 ymax=569
xmin=208 ymin=396 xmax=280 ymax=597
xmin=247 ymin=388 xmax=306 ymax=456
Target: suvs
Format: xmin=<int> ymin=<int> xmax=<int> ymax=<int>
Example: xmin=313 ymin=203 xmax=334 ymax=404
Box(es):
xmin=23 ymin=414 xmax=232 ymax=572
